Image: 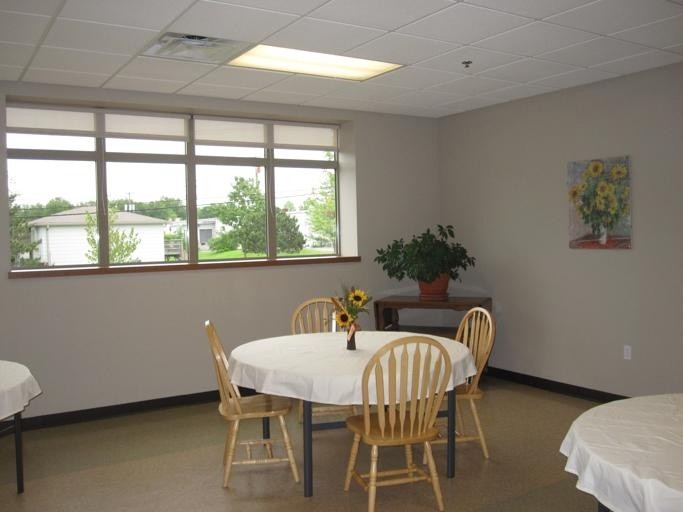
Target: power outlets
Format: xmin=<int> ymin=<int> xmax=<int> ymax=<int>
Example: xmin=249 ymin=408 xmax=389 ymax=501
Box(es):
xmin=624 ymin=344 xmax=631 ymax=360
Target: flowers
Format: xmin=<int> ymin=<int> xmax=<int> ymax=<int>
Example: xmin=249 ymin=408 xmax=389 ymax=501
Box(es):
xmin=321 ymin=287 xmax=374 ymax=326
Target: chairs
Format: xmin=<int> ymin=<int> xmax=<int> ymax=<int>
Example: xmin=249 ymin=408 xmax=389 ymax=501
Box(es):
xmin=415 ymin=307 xmax=496 ymax=466
xmin=289 ymin=299 xmax=361 ymax=432
xmin=343 ymin=335 xmax=454 ymax=510
xmin=200 ymin=318 xmax=302 ymax=490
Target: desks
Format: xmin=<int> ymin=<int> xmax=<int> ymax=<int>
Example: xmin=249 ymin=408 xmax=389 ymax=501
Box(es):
xmin=0 ymin=360 xmax=40 ymax=498
xmin=225 ymin=328 xmax=481 ymax=498
xmin=560 ymin=391 xmax=683 ymax=512
xmin=372 ymin=294 xmax=495 ymax=378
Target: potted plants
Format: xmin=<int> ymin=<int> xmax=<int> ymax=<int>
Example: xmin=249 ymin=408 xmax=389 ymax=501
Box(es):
xmin=374 ymin=222 xmax=479 ymax=296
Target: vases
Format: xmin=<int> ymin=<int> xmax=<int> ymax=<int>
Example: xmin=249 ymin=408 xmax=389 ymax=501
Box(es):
xmin=345 ymin=328 xmax=358 ymax=350
